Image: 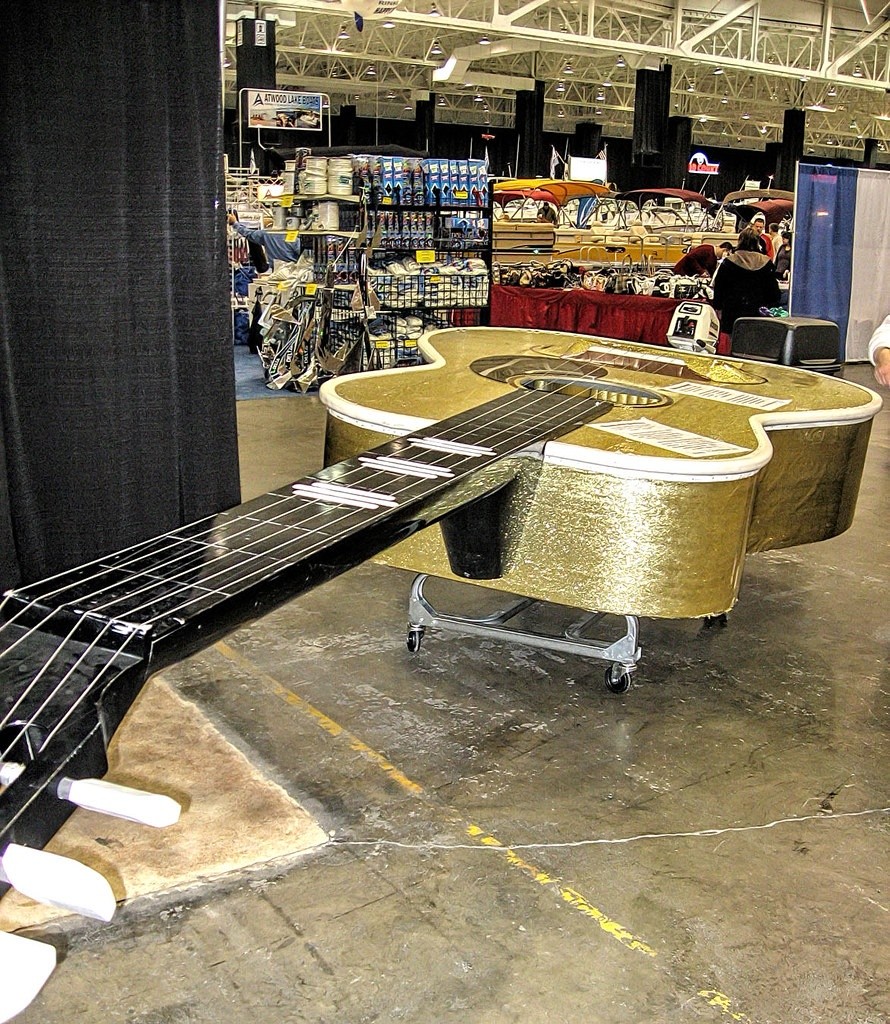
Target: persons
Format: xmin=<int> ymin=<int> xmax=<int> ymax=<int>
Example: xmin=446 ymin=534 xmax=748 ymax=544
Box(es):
xmin=228 ymin=212 xmax=300 ymax=351
xmin=673 ymin=242 xmax=733 ymax=277
xmin=750 ymin=211 xmax=774 ymax=262
xmin=772 ymin=232 xmax=792 ymax=280
xmin=868 ymin=315 xmax=890 ymax=386
xmin=713 ymin=227 xmax=781 ymax=355
xmin=538 ymin=202 xmax=558 ymax=225
xmin=769 ymin=223 xmax=783 ymax=264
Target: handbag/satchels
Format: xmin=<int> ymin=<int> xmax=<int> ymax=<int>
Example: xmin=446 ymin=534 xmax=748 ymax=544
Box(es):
xmin=576 ymin=246 xmax=714 ymax=300
xmin=488 ymin=258 xmax=580 ymax=289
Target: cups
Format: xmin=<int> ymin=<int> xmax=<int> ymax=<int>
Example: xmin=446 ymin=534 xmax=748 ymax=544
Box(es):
xmin=286 ymin=217 xmax=300 ymax=231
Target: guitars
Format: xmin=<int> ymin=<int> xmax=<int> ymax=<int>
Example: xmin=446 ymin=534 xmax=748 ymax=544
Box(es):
xmin=0 ymin=325 xmax=890 ymax=1024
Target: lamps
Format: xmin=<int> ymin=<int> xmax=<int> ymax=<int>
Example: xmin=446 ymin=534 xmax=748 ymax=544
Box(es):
xmin=337 ymin=23 xmax=890 ymax=151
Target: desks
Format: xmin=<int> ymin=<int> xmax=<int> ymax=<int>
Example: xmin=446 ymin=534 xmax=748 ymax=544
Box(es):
xmin=489 ymin=284 xmax=708 ymax=349
